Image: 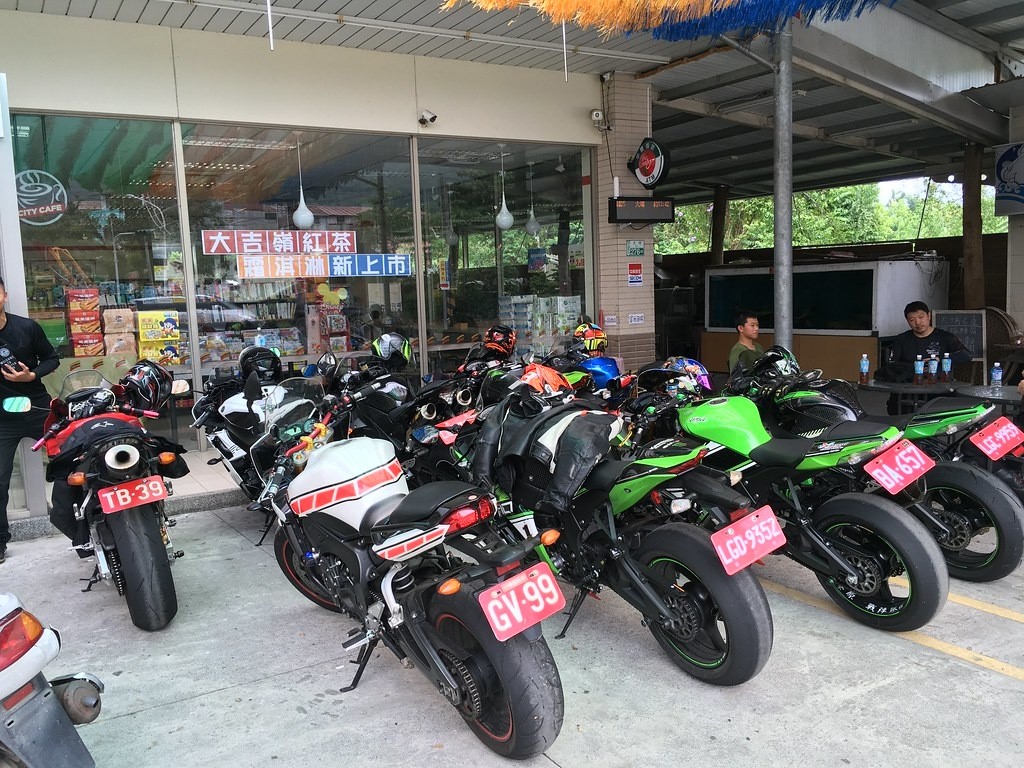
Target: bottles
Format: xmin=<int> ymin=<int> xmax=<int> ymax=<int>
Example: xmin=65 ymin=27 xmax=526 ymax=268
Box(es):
xmin=254 ymin=327 xmax=265 ymax=347
xmin=989 ymin=362 xmax=1003 ymax=397
xmin=941 ymin=353 xmax=951 ymax=382
xmin=913 ymin=354 xmax=925 ymax=385
xmin=927 ymin=355 xmax=938 ymax=384
xmin=859 ymin=353 xmax=870 ymax=384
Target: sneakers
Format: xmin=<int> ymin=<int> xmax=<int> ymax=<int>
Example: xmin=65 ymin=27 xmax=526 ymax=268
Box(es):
xmin=0 ymin=539 xmax=7 ymax=562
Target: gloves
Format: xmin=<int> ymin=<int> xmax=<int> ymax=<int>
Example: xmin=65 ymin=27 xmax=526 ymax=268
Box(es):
xmin=508 ymin=383 xmax=574 ymax=418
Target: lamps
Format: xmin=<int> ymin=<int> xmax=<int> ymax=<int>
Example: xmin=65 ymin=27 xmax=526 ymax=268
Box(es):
xmin=495 ymin=143 xmax=514 ymax=229
xmin=292 ymin=130 xmax=314 ymax=230
xmin=445 ymin=178 xmax=459 ymax=245
xmin=525 ymin=161 xmax=540 ymax=235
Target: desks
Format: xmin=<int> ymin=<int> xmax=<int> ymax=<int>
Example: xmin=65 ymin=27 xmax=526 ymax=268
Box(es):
xmin=859 ymin=379 xmax=969 ymax=415
xmin=956 ymin=385 xmax=1024 ymax=423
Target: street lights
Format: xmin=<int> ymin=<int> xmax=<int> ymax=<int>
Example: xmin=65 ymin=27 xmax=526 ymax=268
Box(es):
xmin=125 ymin=194 xmax=167 ymax=295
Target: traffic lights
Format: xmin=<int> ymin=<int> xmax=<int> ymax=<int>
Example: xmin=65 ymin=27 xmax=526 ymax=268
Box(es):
xmin=134 ymin=229 xmax=151 ymax=236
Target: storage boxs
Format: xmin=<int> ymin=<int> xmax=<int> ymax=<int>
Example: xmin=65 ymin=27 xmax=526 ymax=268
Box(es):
xmin=63 ymin=289 xmax=180 ymax=367
xmin=497 ymin=294 xmax=581 ymax=364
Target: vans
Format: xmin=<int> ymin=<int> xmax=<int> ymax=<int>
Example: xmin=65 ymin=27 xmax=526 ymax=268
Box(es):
xmin=132 ymin=295 xmax=257 ymax=323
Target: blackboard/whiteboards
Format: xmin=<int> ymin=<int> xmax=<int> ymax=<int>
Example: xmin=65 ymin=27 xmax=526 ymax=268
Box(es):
xmin=932 ymin=309 xmax=987 ymax=362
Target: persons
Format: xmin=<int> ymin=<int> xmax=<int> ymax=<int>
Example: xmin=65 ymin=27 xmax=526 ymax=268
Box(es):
xmin=729 ymin=310 xmax=765 ymax=375
xmin=0 ymin=275 xmax=60 ymax=564
xmin=886 ymin=301 xmax=973 ymax=415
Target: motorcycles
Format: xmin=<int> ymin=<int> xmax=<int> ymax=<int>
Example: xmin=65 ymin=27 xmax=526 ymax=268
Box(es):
xmin=189 ymin=344 xmax=565 ymax=760
xmin=3 ymin=370 xmax=189 ymax=632
xmin=319 ymin=331 xmax=773 ymax=687
xmin=0 ymin=589 xmax=105 ymax=768
xmin=721 ymin=344 xmax=1024 ymax=582
xmin=525 ymin=319 xmax=949 ymax=634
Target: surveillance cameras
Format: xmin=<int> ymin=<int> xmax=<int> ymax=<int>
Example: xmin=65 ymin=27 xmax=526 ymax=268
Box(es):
xmin=423 ymin=110 xmax=437 ymax=122
xmin=590 ymin=109 xmax=603 ymax=121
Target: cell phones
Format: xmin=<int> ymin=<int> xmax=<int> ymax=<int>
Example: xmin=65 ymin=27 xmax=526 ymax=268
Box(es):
xmin=0 ymin=344 xmax=23 ymax=374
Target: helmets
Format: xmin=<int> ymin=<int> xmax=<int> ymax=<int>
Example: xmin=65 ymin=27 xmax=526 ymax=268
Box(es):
xmin=573 ymin=322 xmax=608 ymax=355
xmin=238 ymin=346 xmax=282 ymax=380
xmin=753 ymin=345 xmax=801 ymax=376
xmin=663 ymin=356 xmax=715 ymax=396
xmin=120 ymin=356 xmax=173 ymax=411
xmin=370 ymin=332 xmax=416 ymax=370
xmin=484 ymin=323 xmax=515 ymax=358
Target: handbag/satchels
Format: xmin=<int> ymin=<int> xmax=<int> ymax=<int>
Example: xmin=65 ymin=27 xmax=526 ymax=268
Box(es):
xmin=873 ymin=361 xmax=914 ymax=383
